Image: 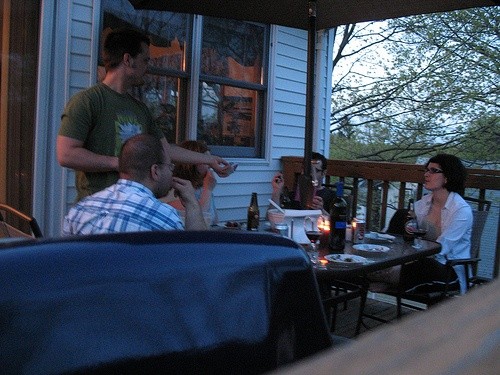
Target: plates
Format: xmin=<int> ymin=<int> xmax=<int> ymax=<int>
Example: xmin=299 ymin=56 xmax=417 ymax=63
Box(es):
xmin=364 ymin=233 xmax=396 ymax=241
xmin=324 ymin=253 xmax=366 ymax=264
xmin=352 ymin=244 xmax=390 ymax=252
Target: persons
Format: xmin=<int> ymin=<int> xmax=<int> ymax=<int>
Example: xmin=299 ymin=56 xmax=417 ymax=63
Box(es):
xmin=57 ymin=28 xmax=238 ymax=234
xmin=360 ymin=153 xmax=473 ymax=292
xmin=265 ymin=152 xmax=337 ymax=222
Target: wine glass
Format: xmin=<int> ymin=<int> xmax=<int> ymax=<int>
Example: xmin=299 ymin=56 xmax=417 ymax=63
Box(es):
xmin=304 ymin=215 xmax=324 ymax=264
xmin=410 ymin=216 xmax=428 ymax=248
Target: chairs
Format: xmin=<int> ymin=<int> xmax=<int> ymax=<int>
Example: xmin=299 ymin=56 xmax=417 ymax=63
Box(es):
xmin=321 ymin=184 xmax=357 ymax=224
xmin=0 ymin=203 xmax=43 ymax=240
xmin=354 ymin=198 xmax=493 ymax=339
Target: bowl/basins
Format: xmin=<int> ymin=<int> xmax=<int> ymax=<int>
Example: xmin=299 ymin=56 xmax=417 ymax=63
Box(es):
xmin=267 ymin=208 xmax=287 ymax=232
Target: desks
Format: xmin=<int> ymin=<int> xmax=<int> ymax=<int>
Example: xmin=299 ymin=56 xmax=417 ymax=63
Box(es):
xmin=210 ymin=220 xmax=442 ymax=341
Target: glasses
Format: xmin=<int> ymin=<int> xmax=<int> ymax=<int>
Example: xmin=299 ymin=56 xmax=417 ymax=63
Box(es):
xmin=423 ymin=167 xmax=444 ymax=173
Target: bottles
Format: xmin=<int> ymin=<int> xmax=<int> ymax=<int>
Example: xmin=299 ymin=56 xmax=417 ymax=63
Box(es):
xmin=328 ymin=182 xmax=348 ymax=254
xmin=403 ymin=199 xmax=417 ymax=242
xmin=246 ymin=193 xmax=260 ymax=231
